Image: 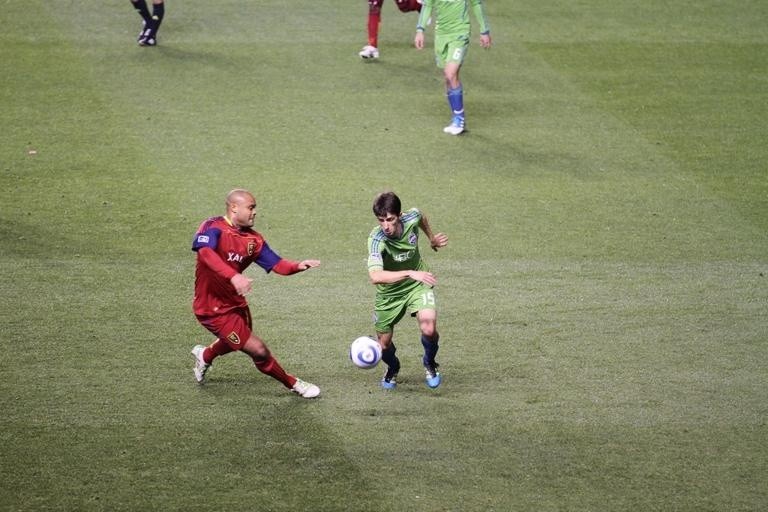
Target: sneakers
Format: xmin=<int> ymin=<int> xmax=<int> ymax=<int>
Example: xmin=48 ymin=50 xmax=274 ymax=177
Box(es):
xmin=382 ymin=359 xmax=400 ymax=389
xmin=359 ymin=45 xmax=379 ymax=59
xmin=290 ymin=378 xmax=320 ymax=398
xmin=443 ymin=109 xmax=465 ymax=135
xmin=137 ymin=29 xmax=156 ymax=46
xmin=424 ymin=357 xmax=440 ymax=388
xmin=191 ymin=345 xmax=212 ymax=383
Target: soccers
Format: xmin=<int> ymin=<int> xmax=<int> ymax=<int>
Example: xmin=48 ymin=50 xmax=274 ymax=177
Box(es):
xmin=348 ymin=336 xmax=382 ymax=368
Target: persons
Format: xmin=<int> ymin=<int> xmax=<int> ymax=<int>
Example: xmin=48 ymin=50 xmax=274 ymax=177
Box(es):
xmin=358 ymin=0 xmax=424 ymax=58
xmin=191 ymin=188 xmax=322 ymax=400
xmin=414 ymin=1 xmax=490 ymax=136
xmin=368 ymin=192 xmax=448 ymax=389
xmin=129 ymin=1 xmax=166 ymax=47
